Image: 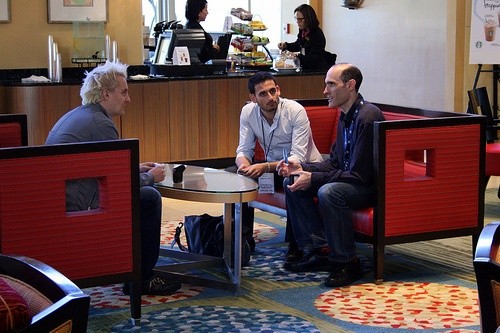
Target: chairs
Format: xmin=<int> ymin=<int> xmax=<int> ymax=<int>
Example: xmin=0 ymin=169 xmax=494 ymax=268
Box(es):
xmin=0 ymin=253 xmax=91 ymax=333
xmin=468 ymin=86 xmax=500 ymax=144
xmin=474 ymin=221 xmax=500 ymax=333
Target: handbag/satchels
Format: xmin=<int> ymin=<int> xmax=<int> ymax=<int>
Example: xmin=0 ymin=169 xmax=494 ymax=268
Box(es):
xmin=298 ymin=48 xmax=337 ymax=73
xmin=172 ymin=213 xmax=252 ymax=269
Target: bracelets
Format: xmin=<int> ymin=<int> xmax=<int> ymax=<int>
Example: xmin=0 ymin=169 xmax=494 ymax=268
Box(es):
xmin=267 ymin=162 xmax=271 ymax=173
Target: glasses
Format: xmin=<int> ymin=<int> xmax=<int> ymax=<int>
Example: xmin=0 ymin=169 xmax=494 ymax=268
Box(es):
xmin=296 ymin=17 xmax=305 ymax=23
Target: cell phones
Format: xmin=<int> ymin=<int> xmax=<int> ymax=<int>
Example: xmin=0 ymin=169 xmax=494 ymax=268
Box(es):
xmin=290 ymin=174 xmax=300 ymax=186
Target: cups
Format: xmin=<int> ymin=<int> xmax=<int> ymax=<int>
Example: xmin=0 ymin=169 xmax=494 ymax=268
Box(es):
xmin=105 ymin=34 xmax=117 ymax=63
xmin=48 ymin=35 xmax=63 ymax=82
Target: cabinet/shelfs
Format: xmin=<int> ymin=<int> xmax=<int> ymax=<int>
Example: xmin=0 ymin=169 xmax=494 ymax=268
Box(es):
xmin=230 ymin=7 xmax=273 ymax=68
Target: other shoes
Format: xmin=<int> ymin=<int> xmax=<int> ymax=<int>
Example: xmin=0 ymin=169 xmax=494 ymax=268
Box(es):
xmin=122 ymin=272 xmax=181 ymax=295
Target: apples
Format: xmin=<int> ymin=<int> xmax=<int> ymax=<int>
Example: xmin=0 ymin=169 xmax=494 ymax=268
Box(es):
xmin=252 ymin=36 xmax=268 ymax=42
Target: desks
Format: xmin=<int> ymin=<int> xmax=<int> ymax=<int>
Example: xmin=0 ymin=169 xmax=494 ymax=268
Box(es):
xmin=144 ymin=160 xmax=260 ymax=294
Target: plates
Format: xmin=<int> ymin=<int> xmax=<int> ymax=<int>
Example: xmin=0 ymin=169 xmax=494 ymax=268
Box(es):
xmin=270 ymin=67 xmax=302 ymax=73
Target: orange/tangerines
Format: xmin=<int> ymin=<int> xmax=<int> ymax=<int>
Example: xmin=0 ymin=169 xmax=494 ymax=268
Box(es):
xmin=245 ymin=52 xmax=265 ymax=57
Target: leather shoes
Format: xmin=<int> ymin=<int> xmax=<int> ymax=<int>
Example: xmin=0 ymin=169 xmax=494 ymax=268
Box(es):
xmin=284 ymin=255 xmax=330 ymax=272
xmin=325 ymin=258 xmax=365 ymax=287
xmin=284 ymin=243 xmax=303 ymax=262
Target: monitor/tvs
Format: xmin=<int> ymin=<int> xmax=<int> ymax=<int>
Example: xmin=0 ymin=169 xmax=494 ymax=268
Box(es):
xmin=168 ymin=30 xmax=206 ymax=58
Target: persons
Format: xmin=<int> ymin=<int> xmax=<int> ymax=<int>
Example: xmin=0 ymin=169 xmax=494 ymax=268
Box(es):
xmin=185 ymin=0 xmax=221 ymax=63
xmin=44 ymin=61 xmax=182 ymax=295
xmin=278 ymin=4 xmax=329 ymax=72
xmin=224 ymin=72 xmax=323 ymax=261
xmin=276 ymin=62 xmax=387 ymax=287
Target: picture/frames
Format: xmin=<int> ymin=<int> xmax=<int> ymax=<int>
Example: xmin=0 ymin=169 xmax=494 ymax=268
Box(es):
xmin=47 ymin=0 xmax=109 ymax=23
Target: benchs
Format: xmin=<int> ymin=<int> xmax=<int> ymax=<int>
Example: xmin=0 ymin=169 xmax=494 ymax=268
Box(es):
xmin=0 ymin=110 xmax=143 ymax=325
xmin=244 ymin=98 xmax=487 ymax=289
